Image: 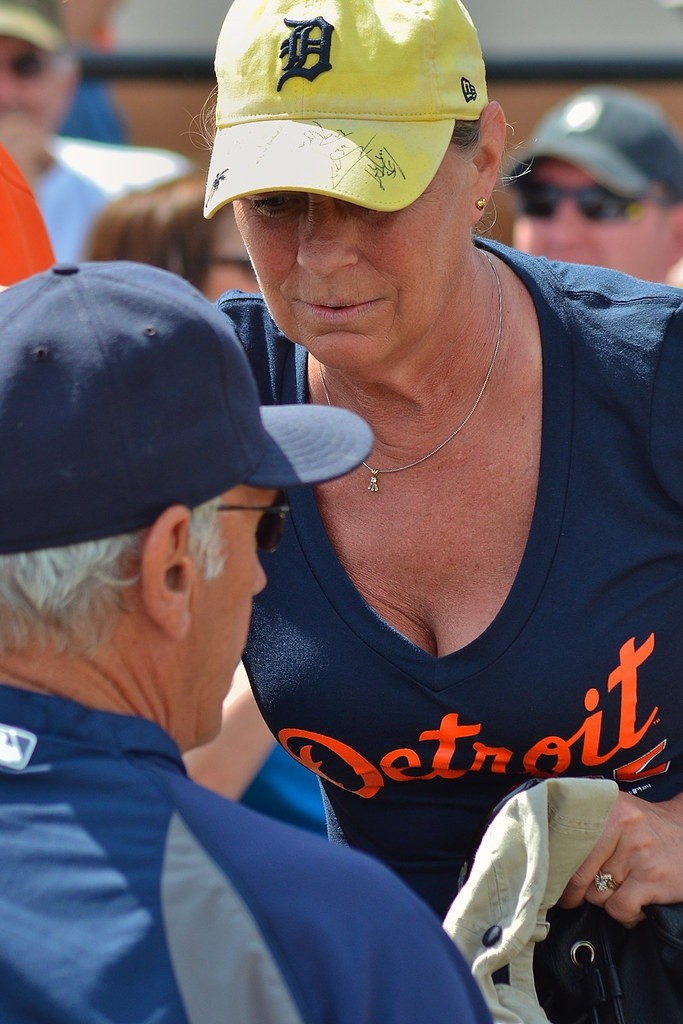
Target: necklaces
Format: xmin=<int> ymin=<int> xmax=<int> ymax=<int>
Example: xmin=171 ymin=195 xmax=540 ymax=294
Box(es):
xmin=316 ymin=247 xmax=502 ymax=492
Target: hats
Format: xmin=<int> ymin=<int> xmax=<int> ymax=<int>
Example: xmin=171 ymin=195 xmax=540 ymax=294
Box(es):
xmin=441 ymin=776 xmax=619 ymax=1023
xmin=0 ymin=0 xmax=69 ymax=54
xmin=501 ymin=84 xmax=683 ymax=206
xmin=0 ymin=259 xmax=374 ymax=556
xmin=202 ymin=0 xmax=490 ymax=220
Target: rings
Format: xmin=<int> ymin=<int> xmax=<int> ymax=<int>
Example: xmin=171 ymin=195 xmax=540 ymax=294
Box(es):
xmin=592 ymin=870 xmax=621 ymax=893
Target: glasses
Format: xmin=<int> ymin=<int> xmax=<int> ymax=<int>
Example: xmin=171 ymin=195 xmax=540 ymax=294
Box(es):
xmin=216 ymin=491 xmax=290 ymax=554
xmin=511 ymin=178 xmax=642 ymax=221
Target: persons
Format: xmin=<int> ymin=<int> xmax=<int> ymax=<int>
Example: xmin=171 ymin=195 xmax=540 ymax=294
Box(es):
xmin=73 ymin=170 xmax=329 ymax=841
xmin=0 ymin=0 xmax=108 ymax=293
xmin=500 ymin=78 xmax=683 ymax=285
xmin=206 ymin=1 xmax=683 ymax=1024
xmin=0 ymin=261 xmax=496 ymax=1023
xmin=54 ymin=0 xmax=126 ymax=144
